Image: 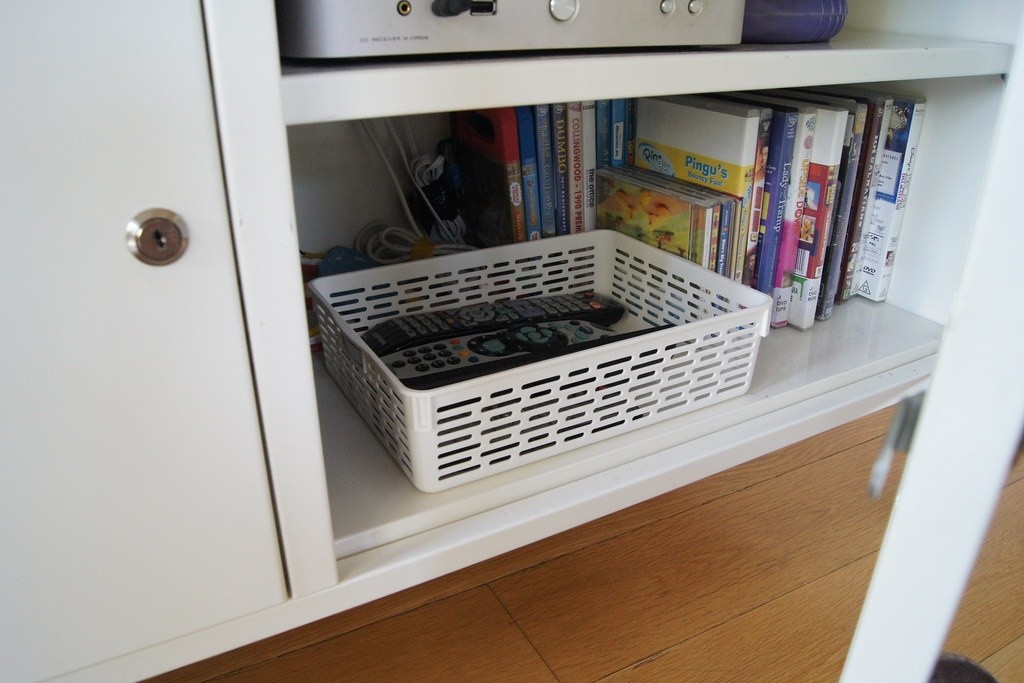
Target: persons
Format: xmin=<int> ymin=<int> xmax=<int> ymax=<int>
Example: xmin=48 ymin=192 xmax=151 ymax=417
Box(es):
xmin=887 ymin=251 xmax=894 ymax=267
xmin=754 ymin=142 xmax=769 ymax=178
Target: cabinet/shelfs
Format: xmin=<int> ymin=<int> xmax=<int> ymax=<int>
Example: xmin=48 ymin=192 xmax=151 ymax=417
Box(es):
xmin=2 ymin=0 xmax=1024 ymax=683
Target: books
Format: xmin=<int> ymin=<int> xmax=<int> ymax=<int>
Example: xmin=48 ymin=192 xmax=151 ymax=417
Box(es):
xmin=451 ymin=89 xmax=925 ymax=334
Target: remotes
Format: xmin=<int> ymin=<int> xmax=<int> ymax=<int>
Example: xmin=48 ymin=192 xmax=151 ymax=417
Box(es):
xmin=361 ymin=290 xmax=625 ymax=355
xmin=380 ymin=320 xmax=619 ymax=391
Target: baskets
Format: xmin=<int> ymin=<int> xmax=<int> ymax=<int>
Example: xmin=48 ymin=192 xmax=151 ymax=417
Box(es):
xmin=306 ymin=227 xmax=774 ymax=494
